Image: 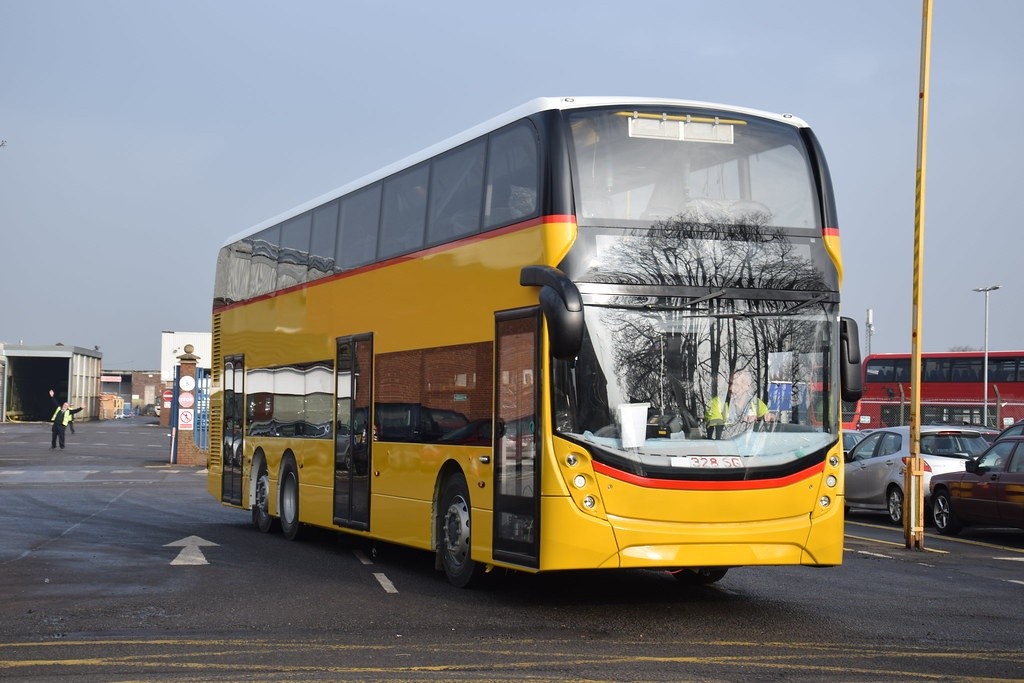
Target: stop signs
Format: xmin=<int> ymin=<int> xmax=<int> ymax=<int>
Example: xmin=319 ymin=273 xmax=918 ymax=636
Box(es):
xmin=162 ymin=390 xmax=173 ymax=402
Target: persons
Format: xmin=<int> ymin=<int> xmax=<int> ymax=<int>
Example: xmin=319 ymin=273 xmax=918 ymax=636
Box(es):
xmin=49 ymin=390 xmax=86 ymax=451
xmin=705 ymin=368 xmax=778 ymax=441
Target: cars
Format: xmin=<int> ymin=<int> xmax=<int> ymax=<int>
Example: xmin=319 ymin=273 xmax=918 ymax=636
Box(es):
xmin=928 ymin=435 xmax=1024 ymax=535
xmin=842 ymin=418 xmax=1024 ymax=524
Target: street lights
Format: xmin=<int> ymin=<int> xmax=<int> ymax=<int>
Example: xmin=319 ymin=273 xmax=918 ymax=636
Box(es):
xmin=971 ymin=285 xmax=1002 ymax=426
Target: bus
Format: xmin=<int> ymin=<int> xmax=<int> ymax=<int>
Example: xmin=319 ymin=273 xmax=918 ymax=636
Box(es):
xmin=206 ymin=94 xmax=865 ymax=588
xmin=804 ymin=363 xmax=863 ymax=431
xmin=858 ymin=349 xmax=1024 ymax=430
